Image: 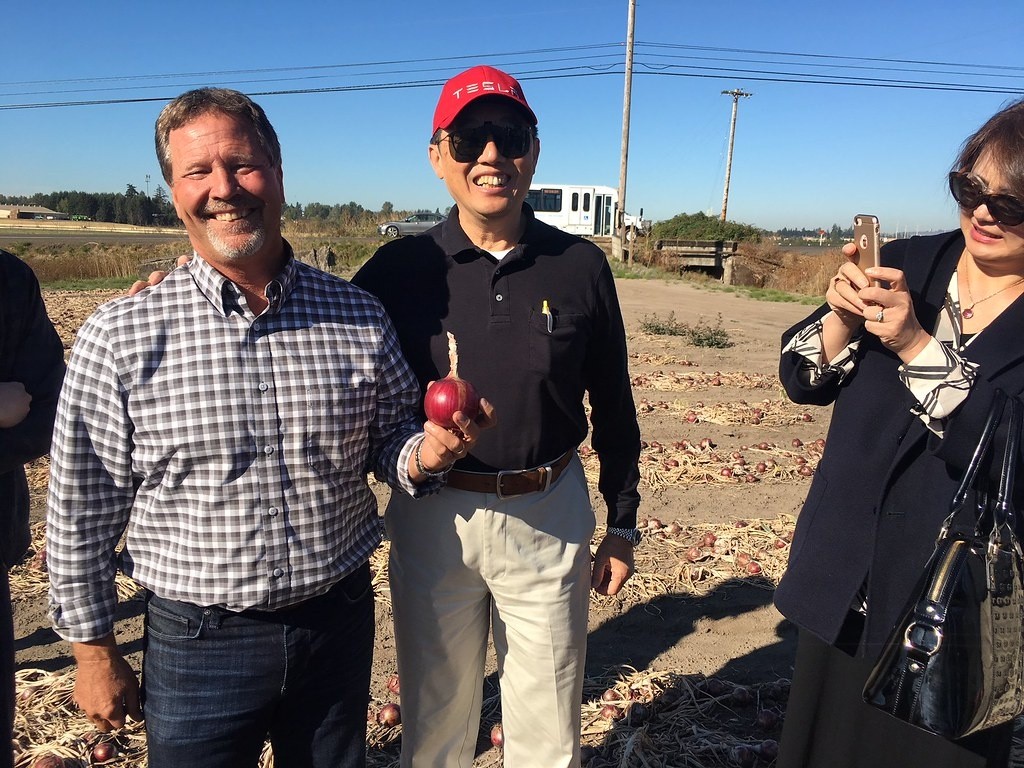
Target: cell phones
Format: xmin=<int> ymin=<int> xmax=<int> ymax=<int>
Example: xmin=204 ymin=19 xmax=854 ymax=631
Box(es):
xmin=851 ymin=214 xmax=881 ymax=308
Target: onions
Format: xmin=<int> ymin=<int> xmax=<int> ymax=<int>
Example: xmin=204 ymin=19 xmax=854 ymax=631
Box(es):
xmin=580 ymin=357 xmax=826 ymax=574
xmin=425 ymin=331 xmax=481 ymax=430
xmin=377 ymin=670 xmax=781 ymax=768
xmin=20 ymin=685 xmax=118 ymax=768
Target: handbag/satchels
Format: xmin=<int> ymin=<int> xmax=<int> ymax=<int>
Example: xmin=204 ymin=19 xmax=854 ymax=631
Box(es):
xmin=863 ymin=386 xmax=1024 ymax=741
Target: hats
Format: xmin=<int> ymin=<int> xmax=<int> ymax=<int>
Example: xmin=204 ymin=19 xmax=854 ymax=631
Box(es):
xmin=432 ymin=66 xmax=538 ymax=136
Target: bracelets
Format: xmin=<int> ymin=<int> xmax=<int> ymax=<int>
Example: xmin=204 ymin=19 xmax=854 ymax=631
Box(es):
xmin=414 ymin=437 xmax=455 ymax=477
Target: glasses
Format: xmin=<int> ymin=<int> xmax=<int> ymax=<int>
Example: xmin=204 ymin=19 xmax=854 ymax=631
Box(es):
xmin=949 ymin=162 xmax=1024 ymax=226
xmin=436 ymin=127 xmax=535 ymax=158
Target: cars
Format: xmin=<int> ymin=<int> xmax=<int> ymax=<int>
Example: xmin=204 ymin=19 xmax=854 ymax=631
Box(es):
xmin=378 ymin=212 xmax=448 ymax=238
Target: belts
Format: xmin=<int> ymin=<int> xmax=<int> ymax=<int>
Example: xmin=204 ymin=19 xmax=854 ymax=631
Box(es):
xmin=448 ymin=447 xmax=573 ymax=500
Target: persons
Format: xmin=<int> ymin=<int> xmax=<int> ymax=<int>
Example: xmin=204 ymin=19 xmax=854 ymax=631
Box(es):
xmin=774 ymin=97 xmax=1023 ymax=768
xmin=0 ymin=249 xmax=67 ymax=768
xmin=46 ymin=87 xmax=498 ymax=768
xmin=127 ymin=66 xmax=641 ymax=768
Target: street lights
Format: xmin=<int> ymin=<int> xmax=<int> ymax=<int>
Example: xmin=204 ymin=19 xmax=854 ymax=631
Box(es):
xmin=722 ymin=86 xmax=753 ymax=225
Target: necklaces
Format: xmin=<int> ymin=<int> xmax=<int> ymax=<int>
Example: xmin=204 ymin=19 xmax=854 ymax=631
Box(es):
xmin=961 ymin=249 xmax=1024 ymax=319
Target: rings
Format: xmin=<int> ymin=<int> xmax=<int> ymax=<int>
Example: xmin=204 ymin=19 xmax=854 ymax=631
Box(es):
xmin=876 ymin=308 xmax=885 ymax=322
xmin=456 ymin=444 xmax=465 ymax=454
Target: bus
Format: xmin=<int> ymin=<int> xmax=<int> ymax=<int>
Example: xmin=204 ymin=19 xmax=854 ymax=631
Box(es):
xmin=520 ymin=182 xmax=649 ymax=242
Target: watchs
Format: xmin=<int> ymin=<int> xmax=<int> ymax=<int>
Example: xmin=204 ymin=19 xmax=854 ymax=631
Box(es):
xmin=606 ymin=526 xmax=641 ymax=546
